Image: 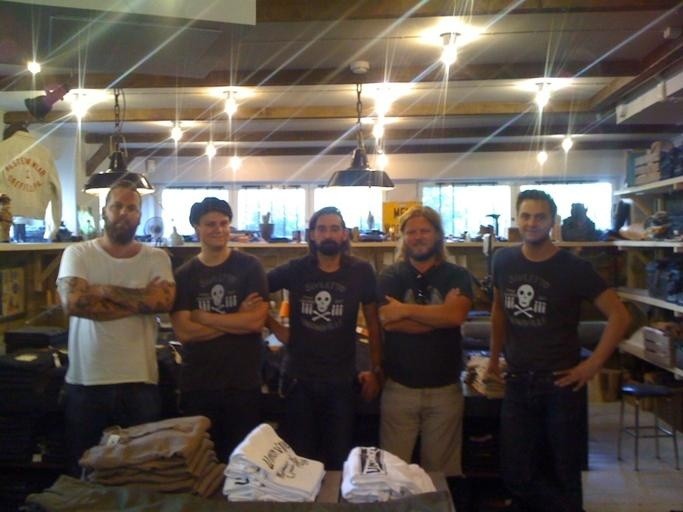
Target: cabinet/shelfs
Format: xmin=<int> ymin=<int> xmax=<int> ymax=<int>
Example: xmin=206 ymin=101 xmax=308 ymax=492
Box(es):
xmin=2 ymin=240 xmax=683 ymax=377
xmin=316 ymin=470 xmax=454 ymax=511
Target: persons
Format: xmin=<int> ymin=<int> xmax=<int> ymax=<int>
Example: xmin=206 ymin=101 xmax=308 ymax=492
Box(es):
xmin=561 ymin=201 xmax=598 ymax=242
xmin=265 ymin=207 xmax=381 ymax=468
xmin=169 ymin=195 xmax=273 ymax=463
xmin=480 ymin=189 xmax=634 ymax=511
xmin=0 ymin=193 xmax=19 ymax=244
xmin=376 ymin=204 xmax=475 ymax=476
xmin=57 ymin=177 xmax=177 ymax=475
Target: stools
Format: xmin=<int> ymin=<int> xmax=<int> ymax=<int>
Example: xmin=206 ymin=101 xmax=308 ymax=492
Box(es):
xmin=618 ymin=383 xmax=679 ymax=473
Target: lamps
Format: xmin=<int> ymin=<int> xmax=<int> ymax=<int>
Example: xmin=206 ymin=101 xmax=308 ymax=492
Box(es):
xmin=24 ymin=74 xmax=78 ymax=121
xmin=82 ymin=87 xmax=157 ymax=195
xmin=440 ymin=33 xmax=460 ymax=67
xmin=534 ymin=82 xmax=551 ymax=109
xmin=325 ymin=57 xmax=396 ymax=193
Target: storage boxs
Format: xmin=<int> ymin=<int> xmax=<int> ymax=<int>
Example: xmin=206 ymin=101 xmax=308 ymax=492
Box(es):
xmin=586 ymin=367 xmax=622 ymax=403
xmin=624 ymin=366 xmax=669 ymax=412
xmin=654 ymin=377 xmax=682 ymax=433
